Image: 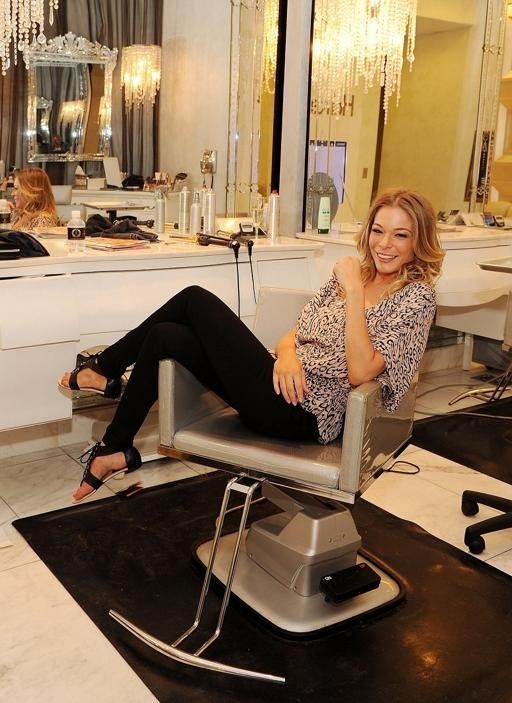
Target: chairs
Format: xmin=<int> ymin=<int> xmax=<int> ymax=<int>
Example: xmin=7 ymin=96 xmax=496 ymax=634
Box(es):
xmin=47 ymin=183 xmax=86 ymax=227
xmin=107 ymin=282 xmax=420 ymax=702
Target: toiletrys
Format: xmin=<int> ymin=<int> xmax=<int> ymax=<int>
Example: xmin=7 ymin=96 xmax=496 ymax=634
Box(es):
xmin=317 ymin=196 xmax=331 ymax=234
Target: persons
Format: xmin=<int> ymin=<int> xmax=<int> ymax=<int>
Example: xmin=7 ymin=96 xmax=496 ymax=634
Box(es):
xmin=8 ymin=166 xmax=68 ymax=231
xmin=61 ymin=184 xmax=447 ymax=500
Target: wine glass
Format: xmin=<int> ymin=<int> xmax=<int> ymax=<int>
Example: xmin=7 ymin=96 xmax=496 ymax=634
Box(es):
xmin=249 ymin=199 xmax=263 ymax=244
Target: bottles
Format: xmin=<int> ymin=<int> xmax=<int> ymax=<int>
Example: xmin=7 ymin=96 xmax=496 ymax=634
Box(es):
xmin=67 ymin=211 xmax=85 ymax=254
xmin=0 ymin=199 xmax=11 ymax=230
xmin=7 ymin=176 xmax=15 ymax=197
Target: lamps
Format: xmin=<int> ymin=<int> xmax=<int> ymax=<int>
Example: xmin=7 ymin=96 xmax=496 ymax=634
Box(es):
xmin=261 ymin=1 xmax=281 ymax=94
xmin=120 ymin=43 xmax=161 ymax=111
xmin=309 ymin=0 xmax=419 ymax=127
xmin=0 ymin=0 xmax=61 ymax=75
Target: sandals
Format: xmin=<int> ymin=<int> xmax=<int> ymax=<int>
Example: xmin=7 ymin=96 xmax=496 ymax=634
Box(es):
xmin=58 ymin=350 xmax=123 ymax=399
xmin=70 ymin=440 xmax=143 ymax=503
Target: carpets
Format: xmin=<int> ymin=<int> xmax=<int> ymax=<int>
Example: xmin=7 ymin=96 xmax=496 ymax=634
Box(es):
xmin=11 ymin=397 xmax=512 ymax=703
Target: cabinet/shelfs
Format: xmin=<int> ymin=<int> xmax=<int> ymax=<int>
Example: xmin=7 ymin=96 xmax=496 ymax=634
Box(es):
xmin=0 ymin=254 xmax=78 ymax=447
xmin=254 ymin=249 xmax=319 ymax=356
xmin=71 ymin=260 xmax=252 ymax=416
xmin=319 ymin=243 xmax=512 ymax=340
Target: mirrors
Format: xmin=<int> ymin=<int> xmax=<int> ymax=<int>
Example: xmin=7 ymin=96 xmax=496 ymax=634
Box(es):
xmin=0 ymin=0 xmax=288 ymax=233
xmin=24 ymin=34 xmax=118 ymax=163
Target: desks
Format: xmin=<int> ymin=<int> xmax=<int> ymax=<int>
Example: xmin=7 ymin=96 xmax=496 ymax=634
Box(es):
xmin=448 ymin=257 xmax=512 ymax=420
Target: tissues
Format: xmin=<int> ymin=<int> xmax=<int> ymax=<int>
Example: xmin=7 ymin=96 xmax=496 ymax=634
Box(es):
xmin=52 ymin=185 xmax=73 ymax=204
